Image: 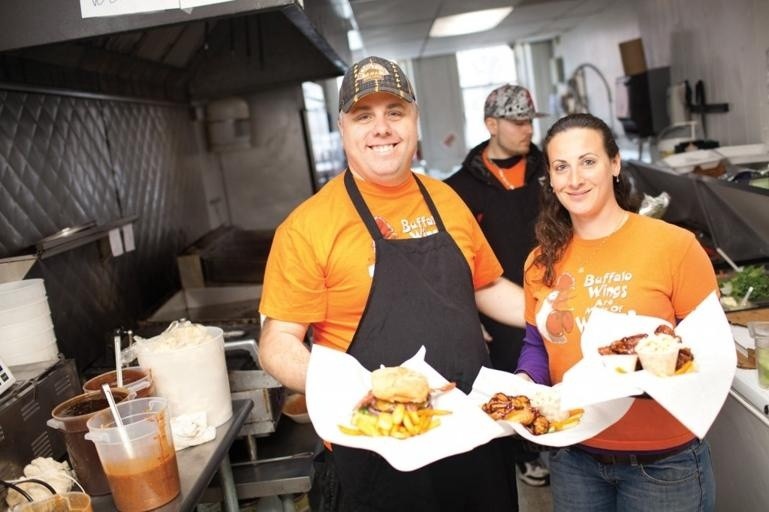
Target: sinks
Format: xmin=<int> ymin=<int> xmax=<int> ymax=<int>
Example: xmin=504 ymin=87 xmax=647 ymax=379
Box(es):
xmin=614 ymin=134 xmax=657 ymax=163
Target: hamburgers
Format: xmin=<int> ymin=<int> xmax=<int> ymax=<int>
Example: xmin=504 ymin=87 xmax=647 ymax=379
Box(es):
xmin=367 ymin=366 xmax=433 ymax=417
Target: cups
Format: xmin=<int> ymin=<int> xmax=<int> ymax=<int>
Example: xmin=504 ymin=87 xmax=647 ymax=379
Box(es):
xmin=14 ymin=489 xmax=94 ymax=512
xmin=751 ymin=320 xmax=769 ymax=389
xmin=49 ymin=325 xmax=232 ymax=512
xmin=635 ymin=346 xmax=679 ymax=378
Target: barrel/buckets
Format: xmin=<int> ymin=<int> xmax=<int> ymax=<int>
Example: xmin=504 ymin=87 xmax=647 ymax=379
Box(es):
xmin=10 ymin=492 xmax=95 ymax=511
xmin=134 ymin=326 xmax=231 ymax=430
xmin=82 ymin=365 xmax=157 ymax=399
xmin=45 ymin=388 xmax=137 ymax=498
xmin=84 ymin=398 xmax=181 ymax=512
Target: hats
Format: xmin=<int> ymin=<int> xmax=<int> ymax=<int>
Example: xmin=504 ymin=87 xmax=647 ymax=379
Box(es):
xmin=338 ymin=56 xmax=416 ymax=113
xmin=484 ymin=85 xmax=549 ymax=123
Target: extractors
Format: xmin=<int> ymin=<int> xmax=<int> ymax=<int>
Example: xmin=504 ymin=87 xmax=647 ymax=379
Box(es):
xmin=1 ymin=0 xmax=354 ymax=107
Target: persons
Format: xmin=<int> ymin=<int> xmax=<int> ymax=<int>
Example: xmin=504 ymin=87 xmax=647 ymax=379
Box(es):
xmin=513 ymin=111 xmax=723 ymax=511
xmin=442 ymin=84 xmax=551 ymax=490
xmin=256 ymin=55 xmax=528 ymax=511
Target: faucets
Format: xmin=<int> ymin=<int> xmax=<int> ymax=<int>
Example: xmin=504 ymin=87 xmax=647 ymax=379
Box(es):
xmin=653 ymin=118 xmax=698 ymax=144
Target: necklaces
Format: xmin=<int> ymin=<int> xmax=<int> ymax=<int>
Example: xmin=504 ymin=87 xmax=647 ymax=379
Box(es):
xmin=484 ymin=149 xmax=516 ymax=190
xmin=568 ymin=209 xmax=629 ymax=255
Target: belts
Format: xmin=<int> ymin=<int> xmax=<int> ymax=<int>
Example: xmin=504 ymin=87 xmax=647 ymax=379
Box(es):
xmin=580 ymin=438 xmax=696 ymax=467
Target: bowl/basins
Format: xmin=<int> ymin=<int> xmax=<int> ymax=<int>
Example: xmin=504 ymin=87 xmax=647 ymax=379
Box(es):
xmin=280 ymin=392 xmax=312 ymax=423
xmin=0 ymin=279 xmax=62 ymax=369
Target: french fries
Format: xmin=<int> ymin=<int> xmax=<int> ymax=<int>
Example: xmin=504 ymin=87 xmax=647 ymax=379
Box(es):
xmin=337 ymin=403 xmax=451 ymax=439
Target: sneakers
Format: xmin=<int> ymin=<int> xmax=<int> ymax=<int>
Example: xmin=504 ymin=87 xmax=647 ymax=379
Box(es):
xmin=515 ymin=459 xmax=550 ymax=488
